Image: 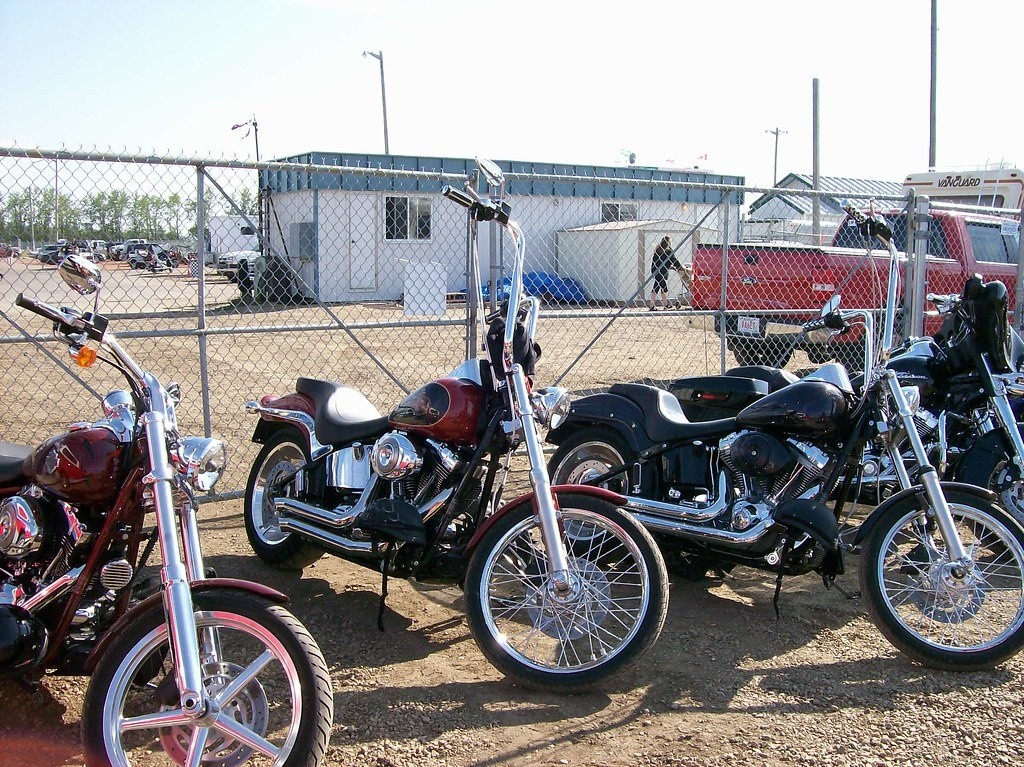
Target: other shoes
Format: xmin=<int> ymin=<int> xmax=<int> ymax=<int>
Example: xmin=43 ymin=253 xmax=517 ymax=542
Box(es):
xmin=649 ymin=306 xmax=657 ymax=311
xmin=664 ymin=305 xmax=672 ymax=309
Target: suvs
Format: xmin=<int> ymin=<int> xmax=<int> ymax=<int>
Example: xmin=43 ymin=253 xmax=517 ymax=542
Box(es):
xmin=217 ymin=242 xmax=261 ymax=283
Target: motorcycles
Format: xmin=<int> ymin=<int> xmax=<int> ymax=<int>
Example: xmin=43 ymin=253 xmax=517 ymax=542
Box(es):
xmin=241 ymin=156 xmax=670 ymax=694
xmin=0 ymin=252 xmax=335 ymax=766
xmin=544 ymin=187 xmax=1024 ymax=676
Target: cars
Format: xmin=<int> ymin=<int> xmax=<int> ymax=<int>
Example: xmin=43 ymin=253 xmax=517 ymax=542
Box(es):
xmin=29 ymin=239 xmax=199 ymax=270
xmin=0 ymin=243 xmax=22 ymax=258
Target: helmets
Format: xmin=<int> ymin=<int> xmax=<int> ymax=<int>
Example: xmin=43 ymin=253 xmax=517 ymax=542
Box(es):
xmin=354 ymin=498 xmax=427 ymax=545
xmin=0 ymin=604 xmax=45 ymax=678
xmin=771 ymin=499 xmax=839 ymax=550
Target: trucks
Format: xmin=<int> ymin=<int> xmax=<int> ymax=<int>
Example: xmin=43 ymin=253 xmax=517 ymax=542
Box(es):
xmin=900 ymin=157 xmax=1024 ymax=223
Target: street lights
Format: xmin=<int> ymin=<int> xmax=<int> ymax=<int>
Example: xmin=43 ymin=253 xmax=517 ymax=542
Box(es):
xmin=231 ymin=113 xmax=264 ymax=257
xmin=764 ymin=127 xmax=789 ymax=187
xmin=361 ymin=49 xmax=390 ymax=155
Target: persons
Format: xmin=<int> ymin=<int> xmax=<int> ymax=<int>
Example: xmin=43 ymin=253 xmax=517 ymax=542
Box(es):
xmin=649 ymin=236 xmax=686 ymax=311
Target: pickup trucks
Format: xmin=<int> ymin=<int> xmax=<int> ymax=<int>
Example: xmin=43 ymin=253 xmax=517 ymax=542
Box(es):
xmin=690 ymin=208 xmax=1024 ymax=375
